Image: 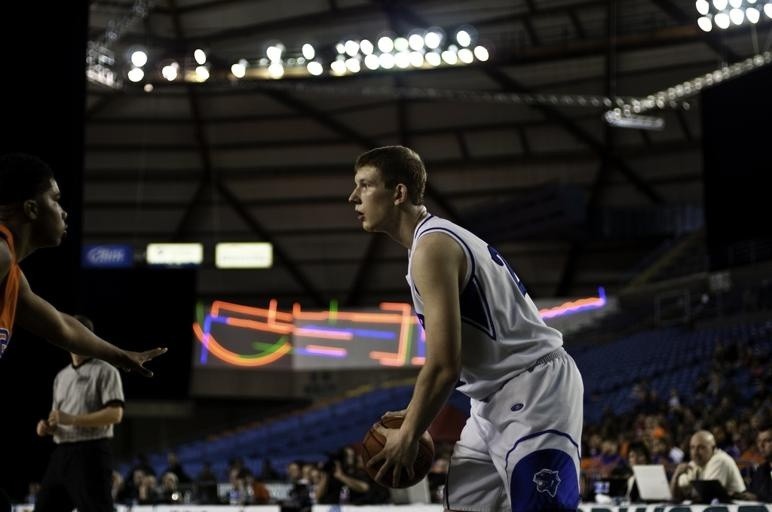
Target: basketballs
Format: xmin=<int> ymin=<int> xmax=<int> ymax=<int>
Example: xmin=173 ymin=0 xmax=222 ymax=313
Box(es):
xmin=361 ymin=416 xmax=435 ymax=488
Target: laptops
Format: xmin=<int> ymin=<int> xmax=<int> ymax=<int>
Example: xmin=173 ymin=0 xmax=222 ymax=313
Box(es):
xmin=632 ymin=464 xmax=682 ymax=502
xmin=690 ymin=480 xmax=732 ymax=504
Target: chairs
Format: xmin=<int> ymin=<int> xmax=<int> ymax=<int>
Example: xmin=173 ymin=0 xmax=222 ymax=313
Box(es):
xmin=111 ymin=319 xmax=763 ymax=492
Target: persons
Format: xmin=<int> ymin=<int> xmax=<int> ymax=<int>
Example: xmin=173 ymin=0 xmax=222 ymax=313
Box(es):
xmin=345 ymin=141 xmax=589 ymax=509
xmin=579 ymin=323 xmax=771 ymax=503
xmin=28 ymin=313 xmax=130 ymax=510
xmin=22 ymin=438 xmax=453 ymax=504
xmin=0 ymin=146 xmax=172 ymax=385
